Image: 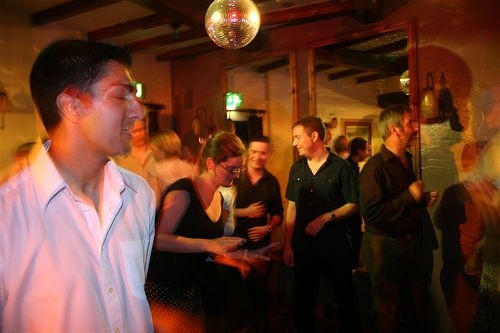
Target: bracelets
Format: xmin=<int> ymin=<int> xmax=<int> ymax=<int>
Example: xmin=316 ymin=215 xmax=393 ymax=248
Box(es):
xmin=330 ymin=211 xmax=335 ymax=220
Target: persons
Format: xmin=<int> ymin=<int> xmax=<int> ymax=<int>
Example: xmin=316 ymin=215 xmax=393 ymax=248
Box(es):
xmin=112 ymin=86 xmax=500 ymax=333
xmin=0 ymin=37 xmax=156 ymax=333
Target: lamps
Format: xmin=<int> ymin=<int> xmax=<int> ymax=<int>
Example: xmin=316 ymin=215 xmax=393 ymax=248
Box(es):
xmin=192 ymin=107 xmax=214 ymax=145
xmin=422 ymin=72 xmax=438 ymax=123
xmin=205 ymin=0 xmax=261 ymax=50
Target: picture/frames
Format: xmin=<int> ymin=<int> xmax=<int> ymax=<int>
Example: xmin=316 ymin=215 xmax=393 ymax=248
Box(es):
xmin=341 ymin=118 xmax=372 ymax=160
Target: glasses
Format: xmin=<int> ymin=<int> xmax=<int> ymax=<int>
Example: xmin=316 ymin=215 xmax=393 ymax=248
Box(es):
xmin=213 ymin=159 xmax=246 ymax=176
